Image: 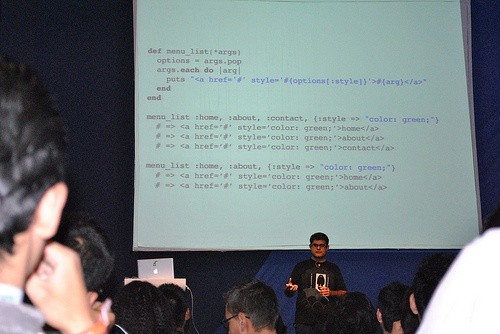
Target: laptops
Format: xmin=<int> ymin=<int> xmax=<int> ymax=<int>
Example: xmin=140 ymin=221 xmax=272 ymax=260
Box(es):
xmin=137 ymin=258 xmax=176 ymax=279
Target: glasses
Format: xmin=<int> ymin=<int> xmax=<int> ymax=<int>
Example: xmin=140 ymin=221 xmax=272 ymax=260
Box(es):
xmin=219 ymin=314 xmax=250 ymax=326
xmin=310 ymin=244 xmax=327 ymax=248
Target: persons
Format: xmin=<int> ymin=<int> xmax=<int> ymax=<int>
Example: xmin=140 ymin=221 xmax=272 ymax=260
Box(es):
xmin=157 ymin=283 xmax=191 ymax=334
xmin=0 ymin=87 xmax=116 ymax=334
xmin=218 ymin=279 xmax=279 ymax=334
xmin=409 ymin=253 xmax=456 ymax=316
xmin=325 ymin=292 xmax=379 ymax=334
xmin=375 ymin=281 xmax=414 ymax=334
xmin=52 ymin=224 xmax=116 ymax=292
xmin=112 ymin=280 xmax=173 ymax=334
xmin=284 ymin=232 xmax=349 ymax=334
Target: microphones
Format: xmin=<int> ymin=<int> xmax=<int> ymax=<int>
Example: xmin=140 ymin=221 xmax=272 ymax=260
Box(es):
xmin=319 ymin=264 xmax=321 ymax=267
